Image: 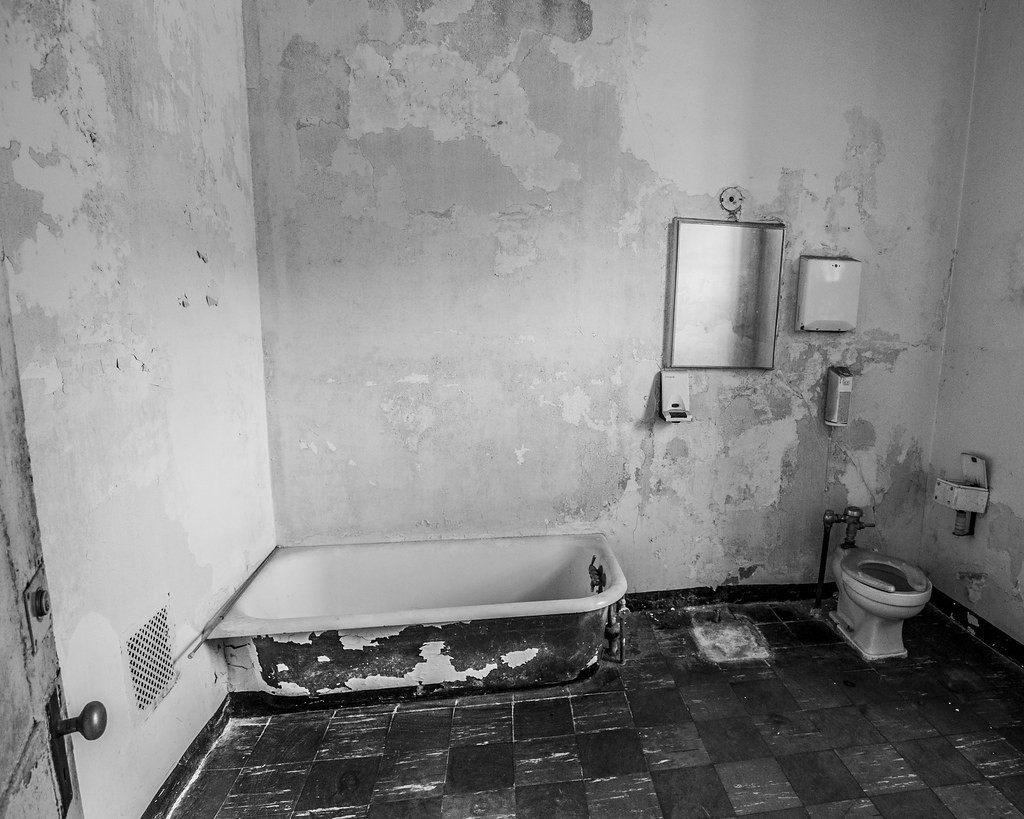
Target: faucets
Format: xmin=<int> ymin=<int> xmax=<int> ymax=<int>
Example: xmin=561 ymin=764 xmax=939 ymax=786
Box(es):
xmin=588 ymin=553 xmax=606 ymax=592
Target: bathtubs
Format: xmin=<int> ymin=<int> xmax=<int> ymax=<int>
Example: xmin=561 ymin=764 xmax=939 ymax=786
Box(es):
xmin=204 ymin=534 xmax=630 ymax=710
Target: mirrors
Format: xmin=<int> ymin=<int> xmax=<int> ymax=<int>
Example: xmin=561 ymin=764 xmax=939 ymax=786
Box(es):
xmin=665 ymin=214 xmax=787 ymax=371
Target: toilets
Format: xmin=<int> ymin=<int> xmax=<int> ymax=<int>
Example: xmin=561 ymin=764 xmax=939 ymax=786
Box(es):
xmin=834 ymin=544 xmax=935 ymax=662
